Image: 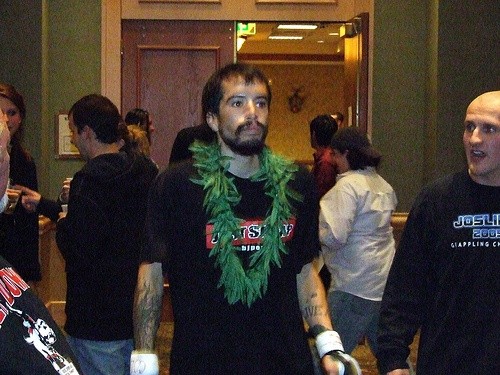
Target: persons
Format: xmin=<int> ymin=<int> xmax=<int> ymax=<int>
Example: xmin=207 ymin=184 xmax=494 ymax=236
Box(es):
xmin=0 ymin=83 xmax=156 ymax=375
xmin=311 ymin=114 xmax=414 ymax=375
xmin=125 ymin=107 xmax=216 ymax=164
xmin=376 ymin=91 xmax=500 ymax=375
xmin=129 ymin=63 xmax=361 ymax=375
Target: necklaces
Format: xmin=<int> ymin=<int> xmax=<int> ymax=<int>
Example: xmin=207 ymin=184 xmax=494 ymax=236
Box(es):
xmin=190 ymin=138 xmax=300 ymax=304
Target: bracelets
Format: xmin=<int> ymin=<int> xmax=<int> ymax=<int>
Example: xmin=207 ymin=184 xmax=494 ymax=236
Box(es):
xmin=315 ymin=331 xmax=345 ymax=358
xmin=129 ymin=354 xmax=159 ymax=375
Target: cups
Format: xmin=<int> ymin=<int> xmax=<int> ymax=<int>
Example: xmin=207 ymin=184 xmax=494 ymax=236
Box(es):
xmin=3 ymin=185 xmax=22 ymax=215
xmin=60 ymin=204 xmax=68 ymax=213
